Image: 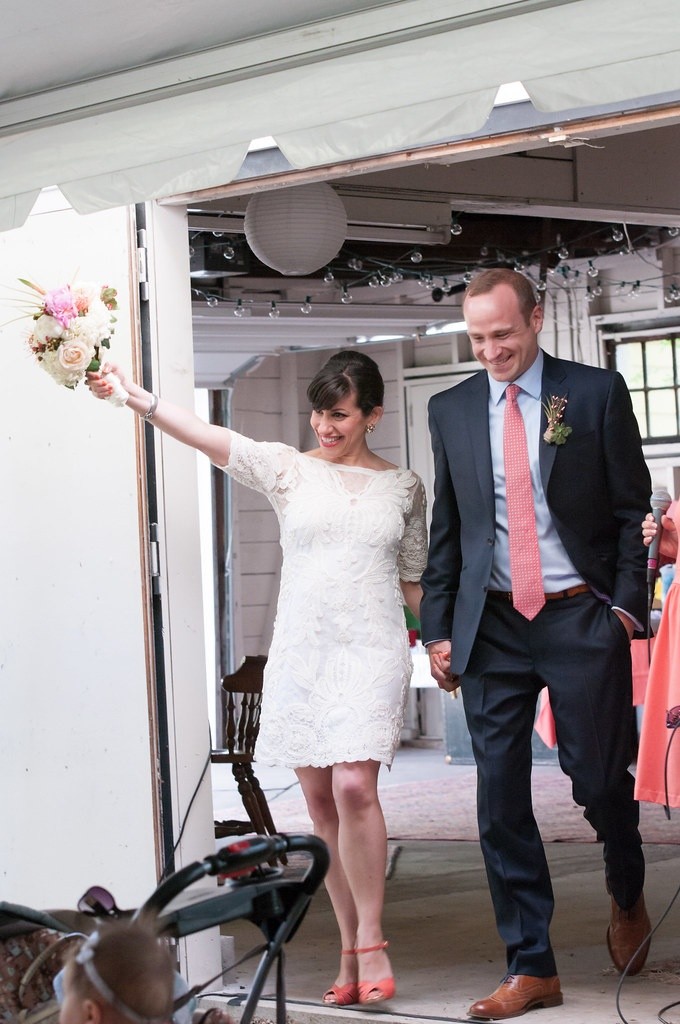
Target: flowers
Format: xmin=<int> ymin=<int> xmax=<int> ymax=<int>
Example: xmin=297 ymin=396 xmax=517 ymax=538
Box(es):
xmin=538 ymin=390 xmax=573 ymax=446
xmin=2 ymin=274 xmax=129 ymax=407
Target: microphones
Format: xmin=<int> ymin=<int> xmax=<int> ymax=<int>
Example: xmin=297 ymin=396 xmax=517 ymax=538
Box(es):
xmin=647 ymin=491 xmax=672 ymax=583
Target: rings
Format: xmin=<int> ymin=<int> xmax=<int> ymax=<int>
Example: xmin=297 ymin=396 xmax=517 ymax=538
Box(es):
xmin=89 ymin=387 xmax=92 ymax=391
xmin=85 ymin=380 xmax=89 ymax=385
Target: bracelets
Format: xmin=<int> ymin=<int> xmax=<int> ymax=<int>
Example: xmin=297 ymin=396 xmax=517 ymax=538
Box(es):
xmin=140 ymin=394 xmax=158 ymax=420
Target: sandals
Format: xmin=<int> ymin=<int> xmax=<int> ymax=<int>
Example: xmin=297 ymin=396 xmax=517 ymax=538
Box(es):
xmin=355 ymin=941 xmax=395 ymax=1004
xmin=321 ymin=948 xmax=358 ymax=1007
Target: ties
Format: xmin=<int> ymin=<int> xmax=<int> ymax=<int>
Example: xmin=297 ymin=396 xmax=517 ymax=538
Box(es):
xmin=503 ymin=384 xmax=546 ymax=621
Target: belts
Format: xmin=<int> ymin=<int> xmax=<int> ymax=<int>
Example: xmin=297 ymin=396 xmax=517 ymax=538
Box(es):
xmin=485 ymin=584 xmax=589 ymax=601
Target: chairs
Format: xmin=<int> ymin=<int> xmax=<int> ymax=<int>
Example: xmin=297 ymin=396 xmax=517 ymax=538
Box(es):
xmin=210 ymin=654 xmax=288 ymax=871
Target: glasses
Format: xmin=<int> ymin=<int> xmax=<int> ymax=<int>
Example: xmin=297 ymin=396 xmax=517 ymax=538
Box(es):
xmin=77 ymin=886 xmax=136 ymax=924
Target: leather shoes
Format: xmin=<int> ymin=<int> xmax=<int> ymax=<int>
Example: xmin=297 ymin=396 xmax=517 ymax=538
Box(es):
xmin=603 ymin=875 xmax=652 ymax=977
xmin=466 ymin=973 xmax=564 ymax=1019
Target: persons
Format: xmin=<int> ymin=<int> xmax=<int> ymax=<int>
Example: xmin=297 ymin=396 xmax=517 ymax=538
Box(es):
xmin=60 ymin=926 xmax=174 ymax=1024
xmin=419 ymin=269 xmax=654 ymax=1016
xmin=93 ymin=349 xmax=458 ymax=1003
xmin=634 ymin=497 xmax=680 ymax=811
